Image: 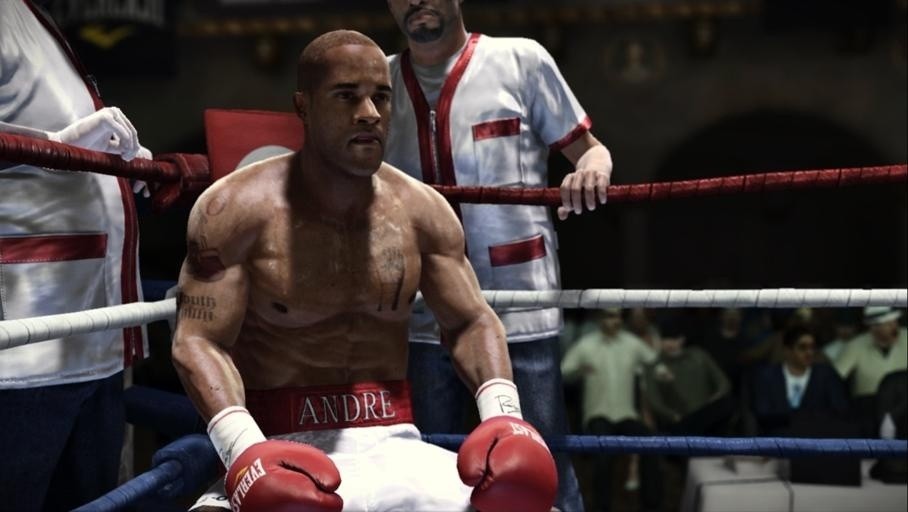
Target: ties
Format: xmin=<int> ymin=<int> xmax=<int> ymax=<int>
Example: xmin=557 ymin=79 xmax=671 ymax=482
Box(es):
xmin=790 ymin=382 xmax=802 ymax=408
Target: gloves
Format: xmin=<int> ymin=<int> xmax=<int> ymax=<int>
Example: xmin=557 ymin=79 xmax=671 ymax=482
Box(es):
xmin=456 ymin=414 xmax=560 ymax=512
xmin=225 ymin=439 xmax=343 ymax=510
xmin=132 ymin=144 xmax=154 ymax=200
xmin=51 ymin=106 xmax=140 ymax=162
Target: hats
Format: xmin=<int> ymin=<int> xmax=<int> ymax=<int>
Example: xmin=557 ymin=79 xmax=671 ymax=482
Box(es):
xmin=861 ymin=304 xmax=902 ymax=324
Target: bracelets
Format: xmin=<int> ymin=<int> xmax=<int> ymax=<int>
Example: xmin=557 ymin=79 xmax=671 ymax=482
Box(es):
xmin=473 ymin=377 xmax=524 ymax=423
xmin=206 ymin=404 xmax=268 ymax=472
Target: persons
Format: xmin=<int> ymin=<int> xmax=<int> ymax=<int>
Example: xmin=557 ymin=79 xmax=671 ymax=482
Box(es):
xmin=0 ymin=0 xmax=153 ymax=512
xmin=372 ymin=0 xmax=615 ymax=511
xmin=162 ymin=28 xmax=562 ymax=511
